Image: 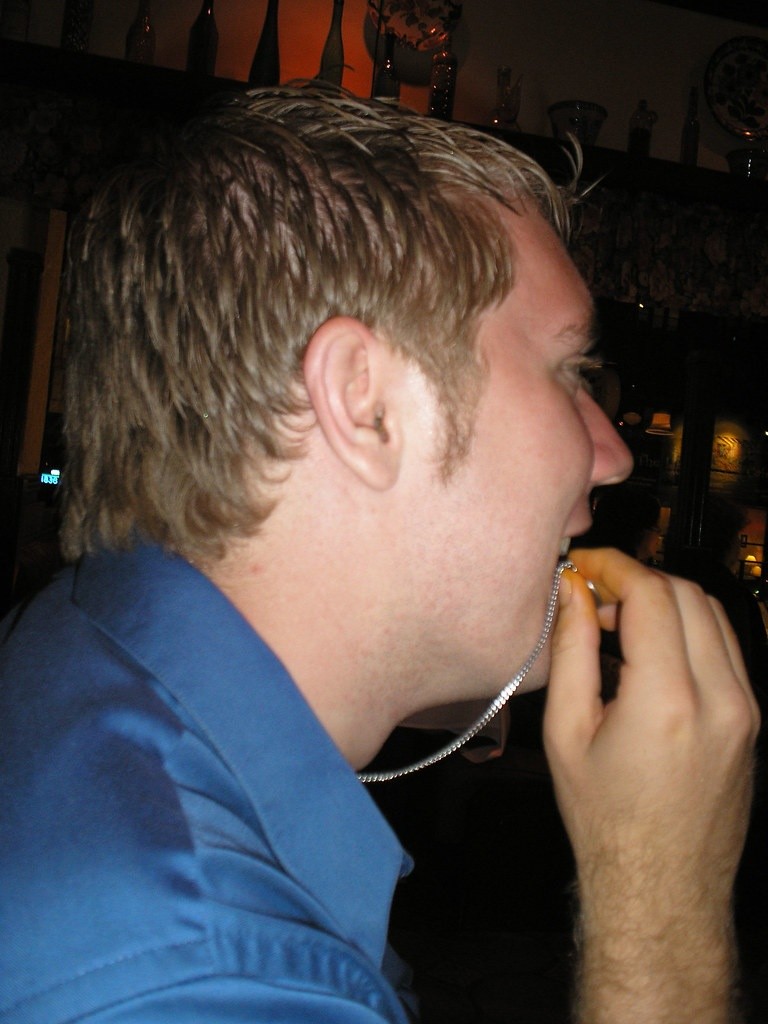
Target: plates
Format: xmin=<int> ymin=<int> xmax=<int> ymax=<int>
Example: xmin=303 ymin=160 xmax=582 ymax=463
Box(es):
xmin=704 ymin=34 xmax=768 ymax=142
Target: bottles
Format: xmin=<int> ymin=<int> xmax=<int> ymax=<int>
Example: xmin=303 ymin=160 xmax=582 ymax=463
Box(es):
xmin=627 ymin=97 xmax=653 ymax=154
xmin=319 ymin=0 xmax=345 ymax=93
xmin=371 ymin=31 xmax=401 ymax=110
xmin=62 ymin=0 xmax=91 ymax=52
xmin=125 ymin=0 xmax=156 ymax=64
xmin=248 ymin=0 xmax=280 ymax=85
xmin=428 ymin=20 xmax=459 ymax=121
xmin=185 ymin=0 xmax=221 ymax=76
xmin=679 ymin=92 xmax=701 ymax=168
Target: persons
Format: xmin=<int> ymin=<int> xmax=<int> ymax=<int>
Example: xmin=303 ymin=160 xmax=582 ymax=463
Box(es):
xmin=660 ymin=493 xmax=768 ymax=1023
xmin=1 ymin=82 xmax=761 ymax=1023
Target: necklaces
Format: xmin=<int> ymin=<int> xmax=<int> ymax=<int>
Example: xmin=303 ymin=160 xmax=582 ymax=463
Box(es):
xmin=358 ymin=556 xmax=601 ymax=784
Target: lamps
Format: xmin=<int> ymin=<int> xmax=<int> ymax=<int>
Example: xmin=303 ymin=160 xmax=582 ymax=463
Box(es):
xmin=645 ymin=411 xmax=676 ymax=436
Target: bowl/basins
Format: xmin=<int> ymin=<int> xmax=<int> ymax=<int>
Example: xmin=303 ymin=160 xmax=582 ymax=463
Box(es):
xmin=546 ymin=101 xmax=609 ymax=147
xmin=724 ymin=147 xmax=768 ymax=181
